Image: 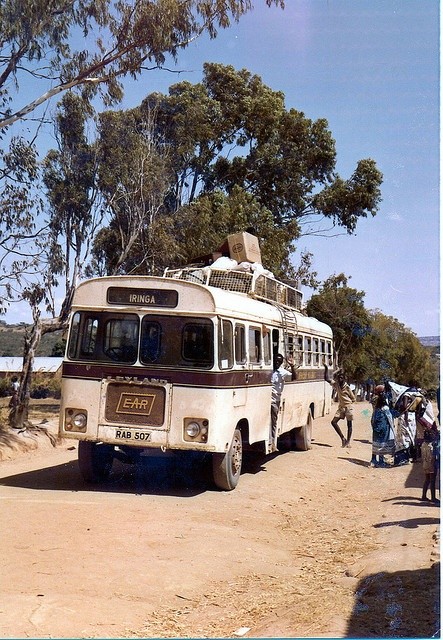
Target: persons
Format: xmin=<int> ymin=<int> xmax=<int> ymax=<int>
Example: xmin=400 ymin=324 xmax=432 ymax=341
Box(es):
xmin=10 ymin=375 xmax=20 ymax=394
xmin=267 ymin=353 xmax=300 ymax=454
xmin=369 ymin=382 xmax=439 ymax=505
xmin=322 ymin=362 xmax=356 ymax=448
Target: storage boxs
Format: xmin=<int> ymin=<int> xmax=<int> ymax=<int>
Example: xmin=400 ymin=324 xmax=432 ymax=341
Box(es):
xmin=226 ymin=231 xmax=261 ymax=264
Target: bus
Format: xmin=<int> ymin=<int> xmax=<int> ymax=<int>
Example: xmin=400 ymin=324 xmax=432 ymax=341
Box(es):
xmin=58 ymin=266 xmax=334 ymax=491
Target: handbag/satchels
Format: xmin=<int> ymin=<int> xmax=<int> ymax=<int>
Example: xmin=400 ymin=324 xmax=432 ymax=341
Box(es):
xmin=172 ymin=266 xmax=203 ymax=283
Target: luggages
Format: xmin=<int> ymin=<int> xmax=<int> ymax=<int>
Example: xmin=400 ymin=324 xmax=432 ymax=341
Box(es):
xmin=191 ymin=252 xmax=222 ymax=267
